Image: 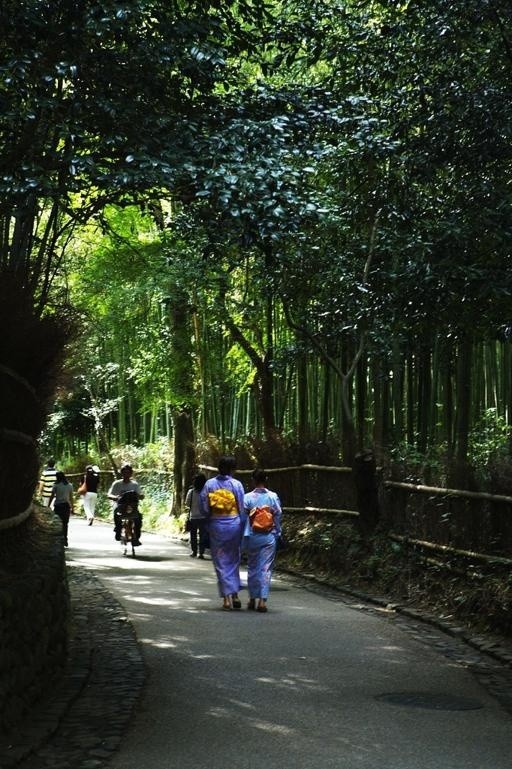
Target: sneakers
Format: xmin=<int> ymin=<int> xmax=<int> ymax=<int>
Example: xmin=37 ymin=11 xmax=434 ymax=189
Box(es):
xmin=189 ymin=554 xmax=204 ymax=559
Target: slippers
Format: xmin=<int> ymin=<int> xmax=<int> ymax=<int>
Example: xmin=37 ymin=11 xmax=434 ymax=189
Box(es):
xmin=248 ymin=602 xmax=267 ymax=612
xmin=223 ymin=601 xmax=241 ymax=611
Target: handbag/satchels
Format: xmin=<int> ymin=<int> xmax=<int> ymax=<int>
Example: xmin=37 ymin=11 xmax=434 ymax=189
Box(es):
xmin=276 ymin=535 xmax=290 ymax=551
xmin=77 ymin=475 xmax=87 ymax=495
xmin=187 ymin=520 xmax=192 ymax=531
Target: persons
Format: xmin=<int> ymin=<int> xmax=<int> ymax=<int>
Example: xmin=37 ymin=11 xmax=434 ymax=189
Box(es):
xmin=38 ymin=459 xmax=57 ymax=511
xmin=106 ymin=464 xmax=146 ymax=545
xmin=78 ymin=464 xmax=101 ymax=526
xmin=183 ymin=472 xmax=208 ymax=559
xmin=47 ymin=470 xmax=75 ymax=548
xmin=242 ymin=468 xmax=283 ymax=612
xmin=198 ymin=456 xmax=245 ymax=610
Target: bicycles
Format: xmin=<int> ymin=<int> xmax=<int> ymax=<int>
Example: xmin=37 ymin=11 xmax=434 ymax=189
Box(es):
xmin=111 ymin=493 xmax=145 ymax=557
